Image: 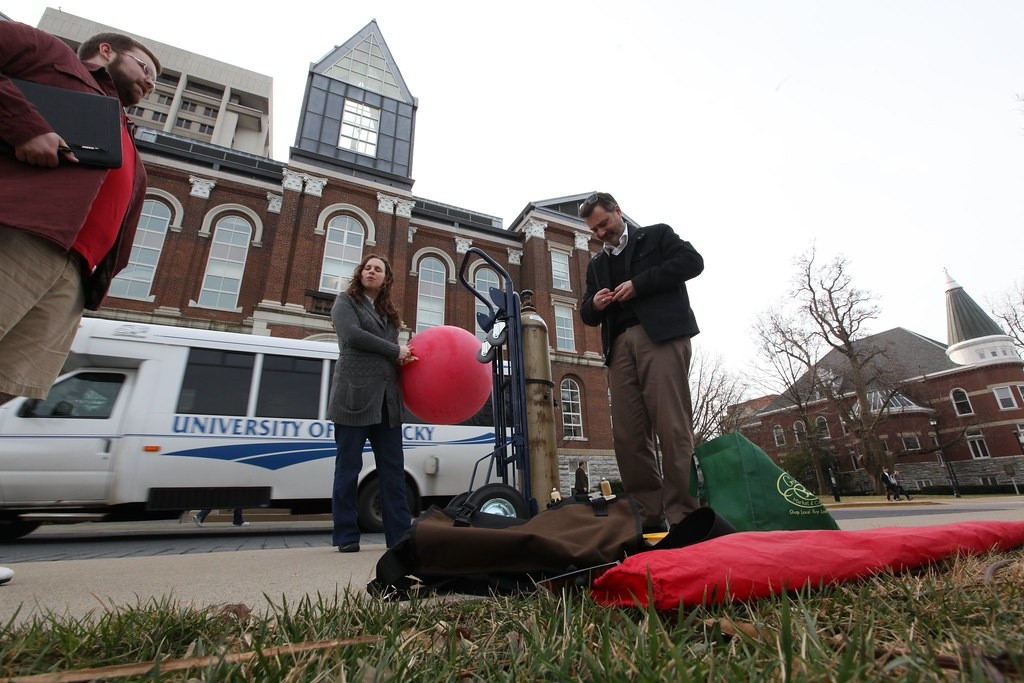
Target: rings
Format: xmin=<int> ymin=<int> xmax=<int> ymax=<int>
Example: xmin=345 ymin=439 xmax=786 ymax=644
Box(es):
xmin=601 ymin=298 xmax=606 ymax=303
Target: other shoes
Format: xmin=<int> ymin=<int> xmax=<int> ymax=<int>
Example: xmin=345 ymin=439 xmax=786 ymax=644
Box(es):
xmin=908 ymin=497 xmax=914 ymax=501
xmin=896 ymin=498 xmax=900 ymax=501
xmin=887 ymin=499 xmax=893 ymax=502
xmin=338 ymin=542 xmax=361 ymax=552
xmin=893 ymin=498 xmax=896 ymax=501
xmin=0 ymin=566 xmax=15 ymax=584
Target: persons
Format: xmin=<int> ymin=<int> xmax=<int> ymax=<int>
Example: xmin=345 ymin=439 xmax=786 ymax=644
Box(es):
xmin=0 ymin=15 xmax=164 ymax=588
xmin=578 ymin=189 xmax=705 ymax=540
xmin=322 ymin=251 xmax=421 ymax=553
xmin=891 ymin=468 xmax=914 ymax=501
xmin=188 ymin=508 xmax=252 ymax=529
xmin=573 ymin=460 xmax=590 ymax=495
xmin=880 ymin=464 xmax=902 ymax=502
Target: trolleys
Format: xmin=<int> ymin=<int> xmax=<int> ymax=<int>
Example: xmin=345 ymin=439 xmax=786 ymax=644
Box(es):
xmin=441 ymin=245 xmax=538 ymax=527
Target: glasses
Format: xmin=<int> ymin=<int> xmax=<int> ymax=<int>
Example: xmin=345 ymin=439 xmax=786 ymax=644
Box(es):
xmin=113 ymin=48 xmax=156 ymax=91
xmin=577 ymin=193 xmax=616 ymax=214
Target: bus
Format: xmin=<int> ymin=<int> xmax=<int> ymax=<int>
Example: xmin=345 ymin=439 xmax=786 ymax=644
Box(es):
xmin=0 ymin=314 xmax=519 ymax=541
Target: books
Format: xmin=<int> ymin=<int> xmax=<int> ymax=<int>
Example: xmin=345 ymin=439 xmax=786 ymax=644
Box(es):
xmin=5 ymin=76 xmax=124 ymax=172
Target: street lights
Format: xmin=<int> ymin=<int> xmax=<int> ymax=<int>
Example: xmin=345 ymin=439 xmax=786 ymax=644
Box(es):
xmin=928 ymin=418 xmax=962 ymax=498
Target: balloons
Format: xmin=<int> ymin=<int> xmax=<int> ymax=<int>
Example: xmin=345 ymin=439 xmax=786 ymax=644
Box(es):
xmin=398 ymin=323 xmax=494 ymax=425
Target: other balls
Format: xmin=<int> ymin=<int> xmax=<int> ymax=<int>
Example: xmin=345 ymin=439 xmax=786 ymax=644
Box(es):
xmin=398 ymin=324 xmax=493 ymax=425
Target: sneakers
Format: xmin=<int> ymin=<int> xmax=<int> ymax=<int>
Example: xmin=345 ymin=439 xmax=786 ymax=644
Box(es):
xmin=232 ymin=521 xmax=250 ymax=527
xmin=192 ymin=515 xmax=204 ymax=528
xmin=900 ymin=498 xmax=904 ymax=500
